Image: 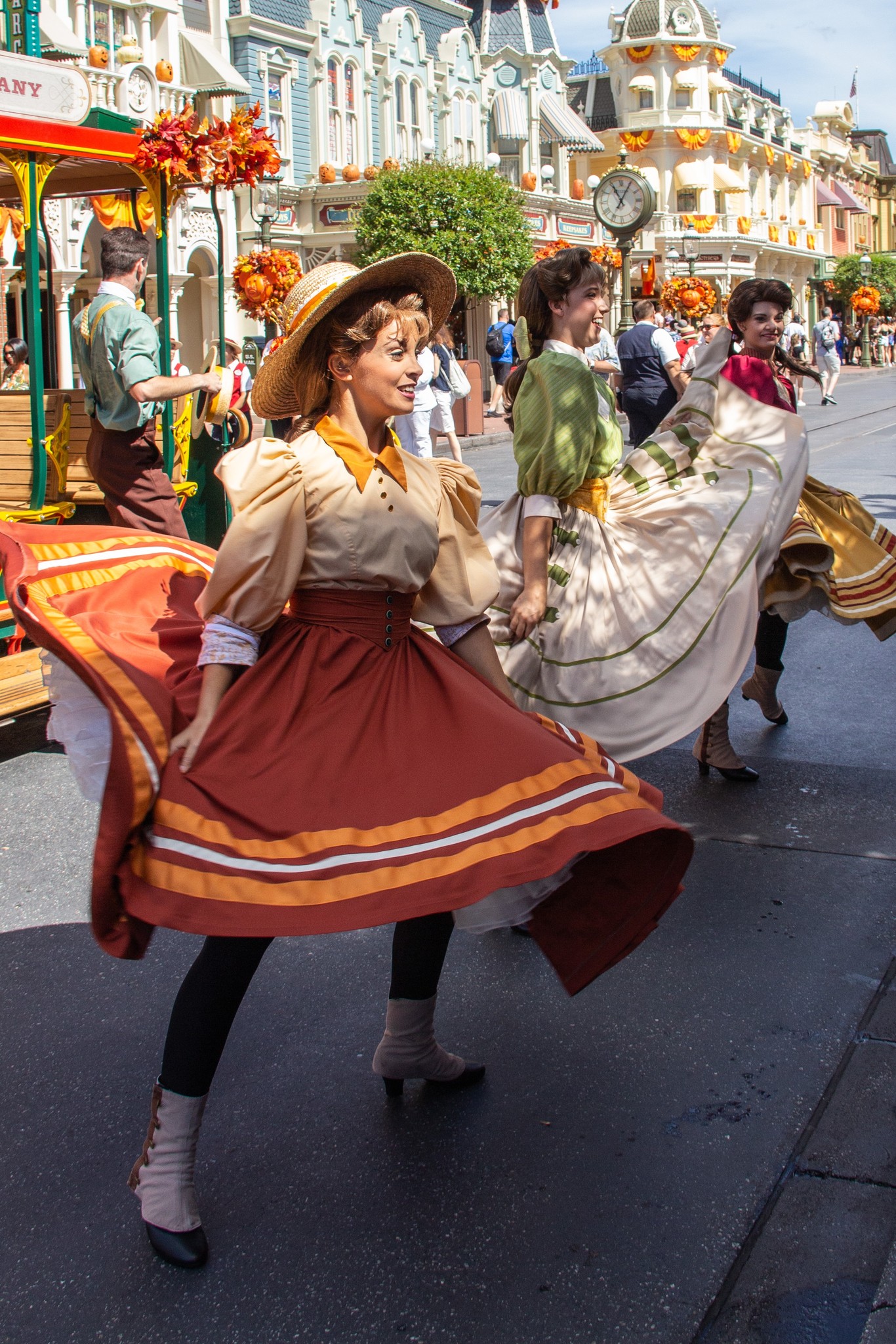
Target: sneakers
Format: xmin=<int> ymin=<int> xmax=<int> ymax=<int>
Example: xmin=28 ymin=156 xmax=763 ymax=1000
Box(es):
xmin=824 ymin=393 xmax=838 ymax=404
xmin=821 ymin=400 xmax=829 ymax=405
xmin=487 ymin=410 xmax=502 ymax=418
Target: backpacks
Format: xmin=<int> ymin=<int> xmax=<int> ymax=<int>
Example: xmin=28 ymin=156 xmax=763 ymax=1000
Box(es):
xmin=820 ymin=322 xmax=835 ymax=346
xmin=486 ymin=324 xmax=507 ymax=356
xmin=438 ymin=343 xmax=471 ymax=399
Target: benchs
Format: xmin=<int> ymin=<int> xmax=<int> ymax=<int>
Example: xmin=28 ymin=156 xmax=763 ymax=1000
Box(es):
xmin=0 ymin=393 xmax=76 ymax=524
xmin=0 ymin=389 xmax=198 ymax=512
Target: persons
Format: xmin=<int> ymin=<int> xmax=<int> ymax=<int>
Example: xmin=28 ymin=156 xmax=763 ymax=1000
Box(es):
xmin=777 ymin=307 xmax=896 ymax=406
xmin=477 ymin=247 xmax=631 ymax=782
xmin=0 ymin=338 xmax=30 ymax=390
xmin=123 ymin=252 xmax=519 ymax=1270
xmin=487 ymin=308 xmax=515 ymax=417
xmin=584 ymin=327 xmax=622 ymax=381
xmin=169 ymin=338 xmax=191 ymax=377
xmin=394 ymin=321 xmax=465 ymax=464
xmin=659 ymin=278 xmax=896 ymax=783
xmin=71 ymin=224 xmax=222 ymax=539
xmin=609 ymin=300 xmax=745 ymax=450
xmin=206 ymin=336 xmax=253 ymax=551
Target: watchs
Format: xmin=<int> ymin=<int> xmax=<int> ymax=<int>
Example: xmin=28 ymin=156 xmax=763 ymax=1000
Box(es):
xmin=590 ymin=360 xmax=595 ymax=371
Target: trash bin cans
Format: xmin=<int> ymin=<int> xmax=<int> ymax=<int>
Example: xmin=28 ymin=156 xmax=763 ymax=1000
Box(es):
xmin=438 ymin=360 xmax=484 ymax=437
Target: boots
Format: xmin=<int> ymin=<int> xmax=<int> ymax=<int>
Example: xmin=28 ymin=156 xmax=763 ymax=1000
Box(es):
xmin=373 ymin=992 xmax=486 ymax=1098
xmin=741 ymin=659 xmax=788 ymax=724
xmin=125 ymin=1075 xmax=209 ymax=1267
xmin=691 ymin=704 xmax=759 ymax=780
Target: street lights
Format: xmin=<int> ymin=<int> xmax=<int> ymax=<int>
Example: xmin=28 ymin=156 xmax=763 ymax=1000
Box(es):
xmin=859 ymin=252 xmax=875 ymax=367
xmin=666 ymin=245 xmax=681 ymax=321
xmin=239 ymin=173 xmax=291 ymax=444
xmin=677 ymin=223 xmax=705 ymax=342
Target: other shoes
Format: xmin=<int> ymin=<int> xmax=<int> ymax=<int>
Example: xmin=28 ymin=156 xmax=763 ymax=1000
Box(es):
xmin=797 ymin=400 xmax=805 ymax=407
xmin=852 ymin=358 xmax=858 ymax=364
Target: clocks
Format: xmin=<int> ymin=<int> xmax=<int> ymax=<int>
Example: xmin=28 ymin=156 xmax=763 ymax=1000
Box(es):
xmin=596 ymin=176 xmax=644 ymax=227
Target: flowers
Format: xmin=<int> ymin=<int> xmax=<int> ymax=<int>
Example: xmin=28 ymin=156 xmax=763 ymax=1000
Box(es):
xmin=590 ymin=244 xmax=622 ymax=270
xmin=533 ymin=240 xmax=576 ymax=261
xmin=850 ymin=286 xmax=880 ymax=314
xmin=133 ymin=99 xmax=283 ymax=189
xmin=662 ymin=276 xmax=717 ymax=317
xmin=231 ymin=249 xmax=302 ymax=319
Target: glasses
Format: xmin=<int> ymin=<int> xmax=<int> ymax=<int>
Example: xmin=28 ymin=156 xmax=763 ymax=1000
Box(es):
xmin=3 ymin=350 xmax=15 ymax=357
xmin=699 ymin=324 xmax=720 ymax=330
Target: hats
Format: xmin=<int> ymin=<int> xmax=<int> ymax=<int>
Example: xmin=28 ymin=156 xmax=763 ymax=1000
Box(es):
xmin=211 ymin=337 xmax=243 ymax=354
xmin=190 ymin=345 xmax=234 ymax=438
xmin=674 ymin=319 xmax=690 ymax=330
xmin=664 ymin=315 xmax=674 ymax=324
xmin=250 ymin=250 xmax=458 ymax=420
xmin=170 ymin=338 xmax=183 ymax=350
xmin=680 ymin=326 xmax=699 ymax=339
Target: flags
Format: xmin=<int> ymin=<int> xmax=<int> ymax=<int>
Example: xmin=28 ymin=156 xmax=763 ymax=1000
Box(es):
xmin=850 ymin=73 xmax=856 ymax=98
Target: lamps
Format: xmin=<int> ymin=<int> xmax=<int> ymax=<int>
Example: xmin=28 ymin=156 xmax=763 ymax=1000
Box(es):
xmin=587 ymin=175 xmax=599 ymax=192
xmin=486 ymin=152 xmax=501 ymax=169
xmin=420 ymin=139 xmax=435 ymax=159
xmin=540 ymin=165 xmax=554 ymax=183
